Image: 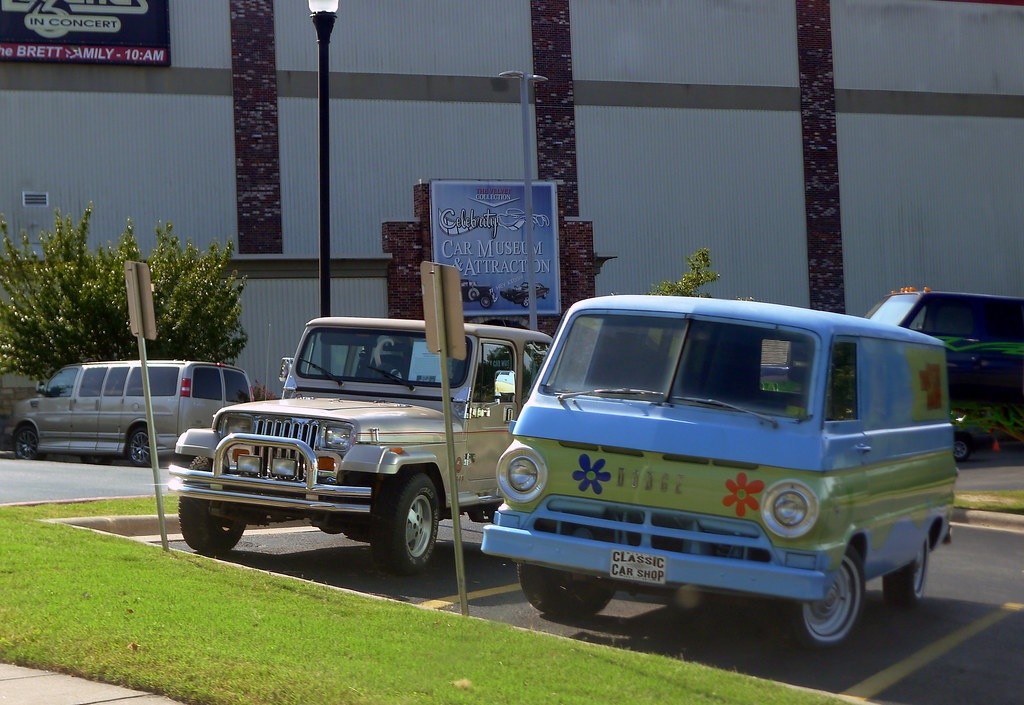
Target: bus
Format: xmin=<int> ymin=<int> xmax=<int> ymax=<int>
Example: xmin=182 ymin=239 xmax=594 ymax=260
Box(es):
xmin=478 ymin=292 xmax=957 ymax=652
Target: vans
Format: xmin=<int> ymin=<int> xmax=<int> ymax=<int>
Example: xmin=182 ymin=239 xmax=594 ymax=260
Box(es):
xmin=11 ymin=360 xmax=257 ymax=467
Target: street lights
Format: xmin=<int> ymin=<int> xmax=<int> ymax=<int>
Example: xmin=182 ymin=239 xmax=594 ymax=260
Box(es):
xmin=500 ymin=70 xmax=551 ymax=331
xmin=307 ymin=0 xmax=340 ymax=376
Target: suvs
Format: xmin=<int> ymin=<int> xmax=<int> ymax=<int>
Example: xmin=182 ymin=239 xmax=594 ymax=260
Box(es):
xmin=165 ymin=315 xmax=555 ymax=576
xmin=862 ymin=286 xmax=1024 ymax=463
